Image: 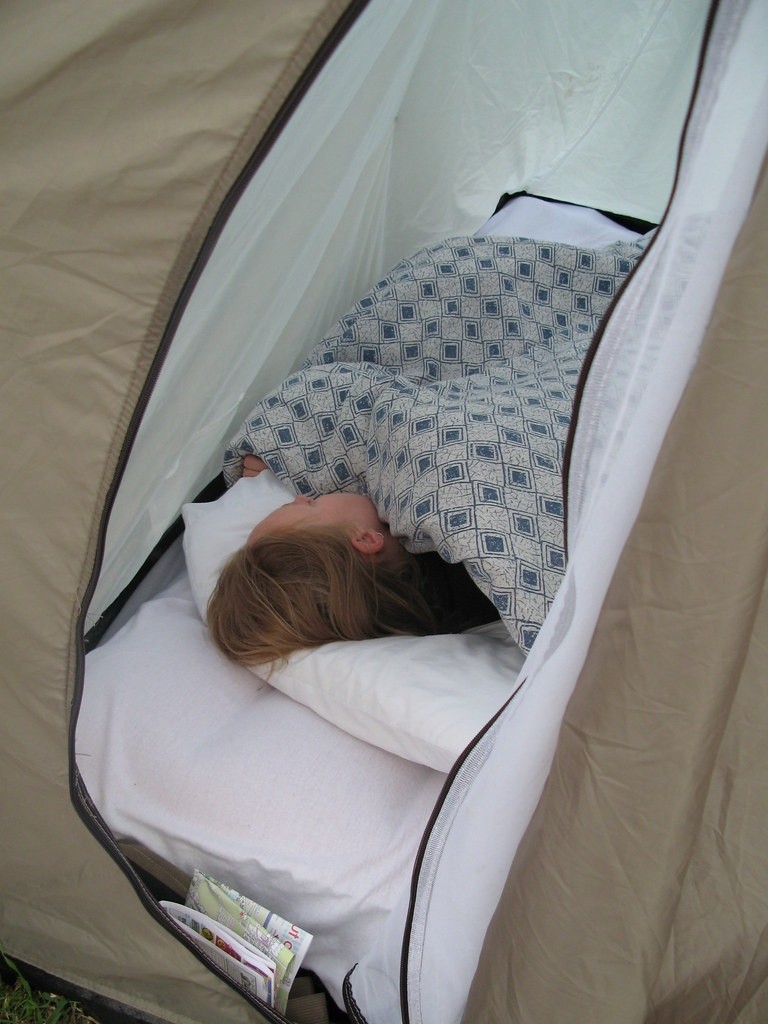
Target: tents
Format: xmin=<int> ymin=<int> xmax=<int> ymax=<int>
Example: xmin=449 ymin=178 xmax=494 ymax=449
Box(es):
xmin=0 ymin=0 xmax=768 ymax=1024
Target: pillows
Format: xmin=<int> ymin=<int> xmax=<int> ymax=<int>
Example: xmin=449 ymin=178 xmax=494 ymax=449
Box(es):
xmin=74 ymin=597 xmax=449 ymax=1010
xmin=179 ymin=470 xmax=525 ymax=776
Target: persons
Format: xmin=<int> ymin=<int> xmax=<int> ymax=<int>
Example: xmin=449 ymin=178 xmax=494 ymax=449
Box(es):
xmin=206 ymin=453 xmax=501 ymax=693
